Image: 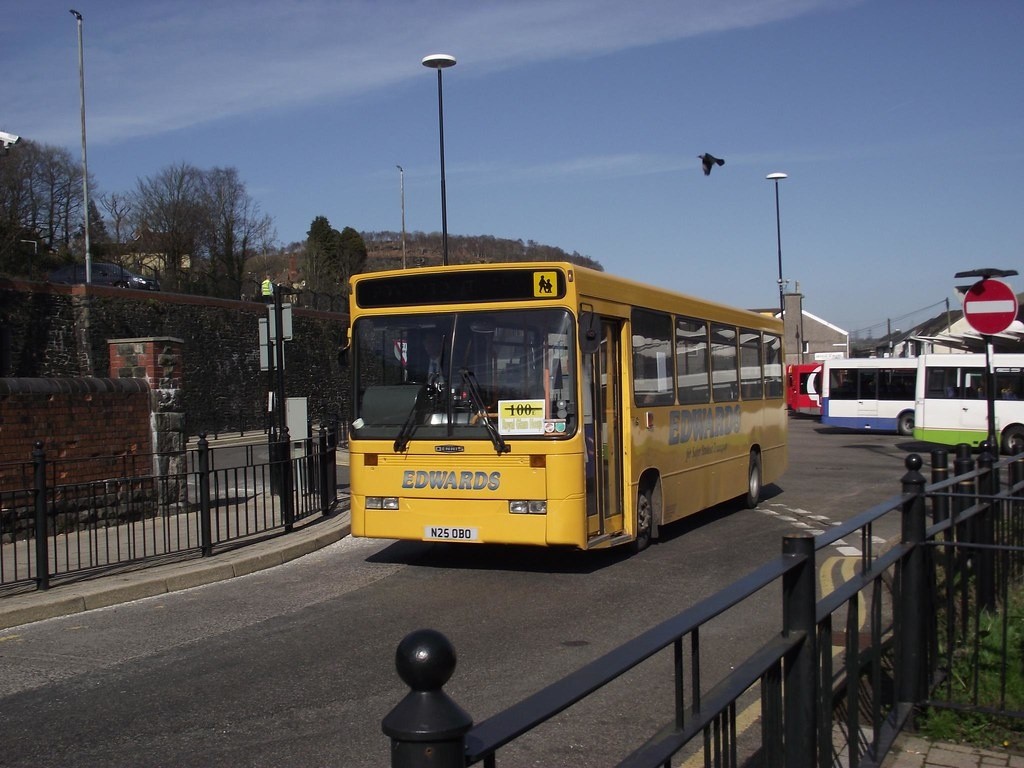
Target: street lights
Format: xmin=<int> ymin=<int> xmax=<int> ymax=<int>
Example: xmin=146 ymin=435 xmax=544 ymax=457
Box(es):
xmin=766 ymin=171 xmax=788 ymax=318
xmin=423 ymin=50 xmax=460 ymax=265
xmin=833 ymin=344 xmax=850 ymax=357
xmin=394 ymin=164 xmax=406 ymax=269
xmin=68 ymin=10 xmax=92 ymax=282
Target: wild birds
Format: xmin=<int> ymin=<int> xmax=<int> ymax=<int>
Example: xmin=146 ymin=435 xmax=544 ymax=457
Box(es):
xmin=696 ymin=152 xmax=725 ymax=176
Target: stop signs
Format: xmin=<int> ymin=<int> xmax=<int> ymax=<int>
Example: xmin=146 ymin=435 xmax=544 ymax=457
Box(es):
xmin=964 ymin=282 xmax=1017 ymax=336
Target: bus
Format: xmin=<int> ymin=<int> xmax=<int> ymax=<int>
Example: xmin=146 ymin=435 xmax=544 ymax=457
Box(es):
xmin=339 ymin=262 xmax=791 ymax=550
xmin=914 ymin=352 xmax=1024 ymax=454
xmin=820 ymin=354 xmax=919 ymax=435
xmin=633 ymin=335 xmax=779 ymax=401
xmin=784 ymin=363 xmax=824 ymax=419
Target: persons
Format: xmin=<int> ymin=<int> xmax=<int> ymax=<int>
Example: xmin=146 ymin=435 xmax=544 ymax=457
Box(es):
xmin=419 ymin=333 xmax=448 ymax=383
xmin=261 ymin=276 xmax=273 ymax=303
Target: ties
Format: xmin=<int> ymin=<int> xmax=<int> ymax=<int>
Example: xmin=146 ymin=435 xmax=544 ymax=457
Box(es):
xmin=432 ymin=362 xmax=437 ymax=384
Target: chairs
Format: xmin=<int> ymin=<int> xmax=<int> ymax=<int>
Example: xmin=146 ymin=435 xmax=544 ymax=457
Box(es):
xmin=947 ymin=386 xmax=956 ymax=398
xmin=1001 ymin=389 xmax=1017 ymax=400
xmin=977 ymin=388 xmax=985 ymax=399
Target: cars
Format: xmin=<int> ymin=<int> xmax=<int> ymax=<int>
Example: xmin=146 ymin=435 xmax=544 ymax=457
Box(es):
xmin=52 ymin=262 xmax=159 ymax=290
